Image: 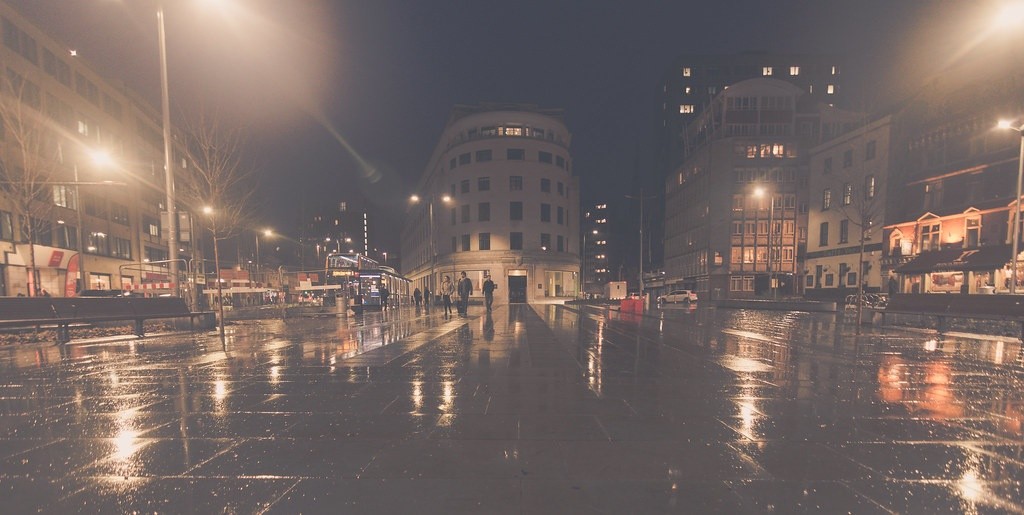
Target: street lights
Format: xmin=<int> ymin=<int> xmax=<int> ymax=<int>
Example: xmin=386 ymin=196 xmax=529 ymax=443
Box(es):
xmin=624 ymin=187 xmax=657 ymax=298
xmin=998 ymin=120 xmax=1024 ymax=294
xmin=752 ymin=186 xmax=766 ymax=271
xmin=74 ymin=149 xmax=114 ymax=291
xmin=256 ymin=227 xmax=272 ymax=270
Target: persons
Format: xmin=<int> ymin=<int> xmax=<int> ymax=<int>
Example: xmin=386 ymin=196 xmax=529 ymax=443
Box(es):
xmin=440 ymin=275 xmax=456 ymax=316
xmin=379 ymin=283 xmax=429 ymax=313
xmin=481 ymin=275 xmax=494 ymax=312
xmin=457 ymin=271 xmax=474 ymax=318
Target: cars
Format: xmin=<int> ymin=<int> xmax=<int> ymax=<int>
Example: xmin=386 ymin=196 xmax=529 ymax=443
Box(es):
xmin=629 ymin=293 xmax=641 ymax=300
xmin=657 ymin=290 xmax=698 ymax=303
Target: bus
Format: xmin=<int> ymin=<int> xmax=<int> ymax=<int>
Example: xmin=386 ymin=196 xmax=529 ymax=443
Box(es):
xmin=325 ymin=252 xmax=379 ymax=305
xmin=378 ymin=265 xmax=400 ymax=288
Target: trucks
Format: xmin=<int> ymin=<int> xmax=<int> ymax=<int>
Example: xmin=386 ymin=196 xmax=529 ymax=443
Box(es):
xmin=604 ymin=281 xmax=627 ymax=303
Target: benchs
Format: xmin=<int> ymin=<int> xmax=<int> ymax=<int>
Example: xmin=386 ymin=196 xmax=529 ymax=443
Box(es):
xmin=843 ymin=291 xmax=891 ymax=319
xmin=0 ymin=296 xmax=216 ymax=346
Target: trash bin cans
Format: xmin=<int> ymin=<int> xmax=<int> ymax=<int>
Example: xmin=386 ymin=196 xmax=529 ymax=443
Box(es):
xmin=335 ymin=292 xmax=347 ymax=314
xmin=619 ymin=299 xmax=644 ymax=315
